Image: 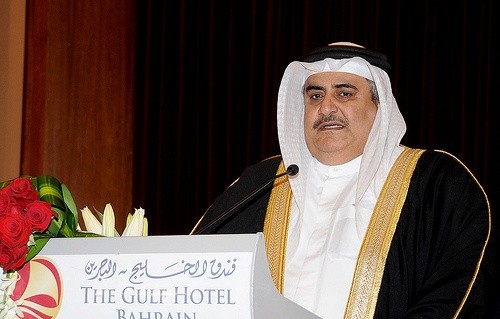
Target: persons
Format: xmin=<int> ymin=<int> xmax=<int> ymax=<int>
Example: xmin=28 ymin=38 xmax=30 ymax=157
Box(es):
xmin=196 ymin=36 xmax=499 ymax=319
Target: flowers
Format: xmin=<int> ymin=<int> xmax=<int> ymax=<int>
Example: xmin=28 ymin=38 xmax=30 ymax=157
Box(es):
xmin=76 ymin=203 xmax=151 ymax=238
xmin=0 ymin=174 xmax=54 ymax=272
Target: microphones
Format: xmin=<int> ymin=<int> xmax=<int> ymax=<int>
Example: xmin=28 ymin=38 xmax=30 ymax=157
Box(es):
xmin=193 ymin=163 xmax=300 ymax=234
